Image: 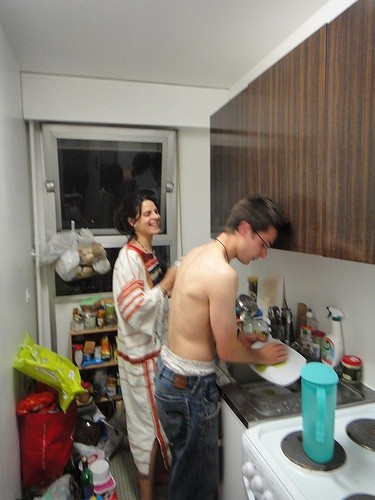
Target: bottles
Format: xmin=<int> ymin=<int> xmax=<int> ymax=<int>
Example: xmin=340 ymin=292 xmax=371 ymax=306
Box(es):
xmin=299 ymin=308 xmax=316 ymax=362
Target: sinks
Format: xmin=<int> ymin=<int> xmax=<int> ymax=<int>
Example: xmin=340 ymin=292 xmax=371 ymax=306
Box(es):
xmin=215 ymin=360 xmax=268 ymax=387
xmin=219 ymin=376 xmax=375 ymax=429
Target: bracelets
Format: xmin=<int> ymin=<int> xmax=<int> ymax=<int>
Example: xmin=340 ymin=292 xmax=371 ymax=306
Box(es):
xmin=158 ymin=282 xmax=168 ymax=294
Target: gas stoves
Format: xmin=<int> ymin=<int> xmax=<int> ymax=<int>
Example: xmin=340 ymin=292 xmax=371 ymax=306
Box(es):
xmin=246 ymin=401 xmax=375 ymax=500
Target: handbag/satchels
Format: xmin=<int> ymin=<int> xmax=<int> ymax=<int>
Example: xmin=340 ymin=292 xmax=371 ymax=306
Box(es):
xmin=19 ymin=380 xmax=124 ymax=500
xmin=12 ymin=331 xmax=88 ymax=414
xmin=39 ymin=220 xmax=111 ymax=282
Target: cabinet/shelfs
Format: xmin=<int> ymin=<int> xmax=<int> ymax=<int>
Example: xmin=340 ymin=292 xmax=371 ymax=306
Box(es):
xmin=210 ymin=0 xmax=375 ymax=264
xmin=68 ymin=326 xmax=123 ymax=402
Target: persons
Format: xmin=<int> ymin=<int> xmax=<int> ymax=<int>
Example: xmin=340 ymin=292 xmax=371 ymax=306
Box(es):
xmin=113 ymin=188 xmax=182 ymax=500
xmin=154 ymin=194 xmax=288 ymax=500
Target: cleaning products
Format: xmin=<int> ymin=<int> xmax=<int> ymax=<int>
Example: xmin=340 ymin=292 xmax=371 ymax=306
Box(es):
xmin=320 ymin=306 xmax=345 ymax=369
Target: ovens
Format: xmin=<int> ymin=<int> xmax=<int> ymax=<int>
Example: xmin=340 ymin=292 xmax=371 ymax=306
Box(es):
xmin=242 ymin=433 xmax=293 ymax=500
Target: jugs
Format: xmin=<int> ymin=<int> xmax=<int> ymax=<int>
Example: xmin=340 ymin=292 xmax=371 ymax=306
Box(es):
xmin=300 ymin=362 xmax=339 ymax=464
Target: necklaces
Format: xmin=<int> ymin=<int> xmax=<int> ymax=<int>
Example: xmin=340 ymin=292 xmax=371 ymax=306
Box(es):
xmin=133 ymin=237 xmax=149 ymax=255
xmin=215 ymin=237 xmax=230 ymax=264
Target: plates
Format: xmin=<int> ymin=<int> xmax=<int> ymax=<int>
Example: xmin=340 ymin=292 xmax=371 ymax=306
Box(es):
xmin=247 ymin=337 xmax=307 ymax=388
